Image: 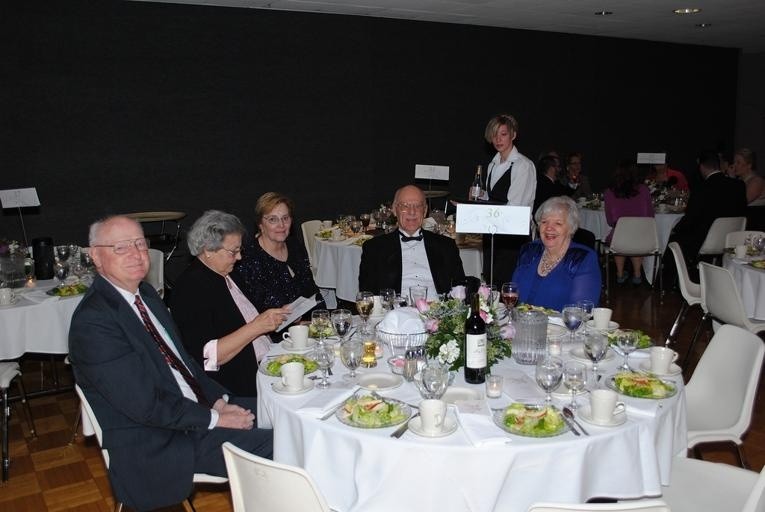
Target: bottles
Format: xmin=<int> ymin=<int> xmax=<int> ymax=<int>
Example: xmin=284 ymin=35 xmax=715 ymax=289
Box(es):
xmin=471 ymin=165 xmax=483 ymax=199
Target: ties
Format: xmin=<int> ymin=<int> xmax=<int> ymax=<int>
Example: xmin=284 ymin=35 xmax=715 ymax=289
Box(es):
xmin=133 ymin=294 xmax=212 ymax=414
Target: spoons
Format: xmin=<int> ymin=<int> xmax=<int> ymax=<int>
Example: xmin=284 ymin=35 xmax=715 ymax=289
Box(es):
xmin=563 ymin=408 xmax=590 ymax=438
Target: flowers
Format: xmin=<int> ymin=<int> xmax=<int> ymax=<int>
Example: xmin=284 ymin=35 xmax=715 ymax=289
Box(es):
xmin=413 ymin=279 xmax=517 ymax=375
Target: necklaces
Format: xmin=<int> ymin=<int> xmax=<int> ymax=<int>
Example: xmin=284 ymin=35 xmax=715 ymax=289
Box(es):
xmin=537 ymin=248 xmax=566 ymax=278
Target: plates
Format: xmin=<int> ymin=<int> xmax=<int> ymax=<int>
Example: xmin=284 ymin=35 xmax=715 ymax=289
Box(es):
xmin=258 ymin=339 xmax=317 ymax=375
xmin=493 ymin=406 xmax=573 ymax=439
xmin=407 ymin=417 xmax=458 ymax=437
xmin=606 ymin=371 xmax=679 ymax=399
xmin=639 ymin=358 xmax=684 ymax=376
xmin=337 ymin=397 xmax=412 ymax=428
xmin=329 ymin=238 xmax=346 ymax=242
xmin=581 ymin=408 xmax=629 ymax=426
xmin=273 ymin=379 xmax=314 ymax=394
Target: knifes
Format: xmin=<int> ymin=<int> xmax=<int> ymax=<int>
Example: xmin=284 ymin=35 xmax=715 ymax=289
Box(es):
xmin=560 ymin=412 xmax=582 ymax=436
xmin=390 ymin=413 xmax=420 ymax=438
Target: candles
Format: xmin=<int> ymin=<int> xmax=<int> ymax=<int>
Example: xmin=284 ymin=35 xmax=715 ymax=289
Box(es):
xmin=487 ymin=382 xmax=501 ymax=397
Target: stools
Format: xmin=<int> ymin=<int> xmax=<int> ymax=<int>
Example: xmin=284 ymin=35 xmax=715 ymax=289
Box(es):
xmin=0 ymin=358 xmax=38 ymax=483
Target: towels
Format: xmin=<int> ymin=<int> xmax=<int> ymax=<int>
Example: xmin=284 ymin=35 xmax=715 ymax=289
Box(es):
xmin=296 ymin=382 xmax=360 ymax=420
xmin=618 ymin=393 xmax=661 ymax=417
xmin=453 ymin=399 xmax=513 ymax=448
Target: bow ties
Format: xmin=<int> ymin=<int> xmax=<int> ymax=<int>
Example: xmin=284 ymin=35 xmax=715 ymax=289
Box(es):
xmin=398 ymin=229 xmax=423 ymax=242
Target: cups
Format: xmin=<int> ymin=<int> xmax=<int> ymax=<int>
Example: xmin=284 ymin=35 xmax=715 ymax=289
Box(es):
xmin=31 ymin=236 xmax=54 ymax=280
xmin=590 ymin=389 xmax=627 ymax=423
xmin=282 ymin=325 xmax=309 ymax=348
xmin=733 ymin=244 xmax=748 ymax=258
xmin=280 ymin=362 xmax=305 ymax=389
xmin=650 ymin=345 xmax=679 ymax=372
xmin=320 ymin=220 xmax=340 ymax=238
xmin=420 ymin=400 xmax=445 ymax=434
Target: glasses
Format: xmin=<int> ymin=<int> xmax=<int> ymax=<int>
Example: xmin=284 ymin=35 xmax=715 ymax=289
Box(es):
xmin=262 ymin=214 xmax=291 ymax=224
xmin=93 ymin=237 xmax=151 ymax=256
xmin=395 ymin=201 xmax=424 ymax=213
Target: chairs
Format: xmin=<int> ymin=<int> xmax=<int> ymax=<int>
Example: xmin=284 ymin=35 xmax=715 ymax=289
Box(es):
xmin=665 ymin=240 xmax=701 ymax=346
xmin=681 ymin=322 xmax=765 ymax=469
xmin=62 ymin=246 xmax=167 ymax=446
xmin=302 ymin=217 xmax=339 ymax=310
xmin=616 ymin=453 xmax=765 ymax=512
xmin=699 ymin=213 xmax=750 ymax=265
xmin=725 ymin=230 xmax=765 ymax=251
xmin=604 ymin=215 xmax=666 ymax=306
xmin=682 ymin=259 xmax=765 ymax=375
xmin=216 ymin=437 xmax=330 ymax=511
xmin=71 ymin=377 xmax=230 ymax=511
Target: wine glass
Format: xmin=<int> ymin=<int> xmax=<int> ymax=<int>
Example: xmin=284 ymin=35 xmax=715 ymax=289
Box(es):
xmin=52 ymin=242 xmax=99 ymax=292
xmin=569 ymin=166 xmax=580 ymax=184
xmin=340 ymin=205 xmax=455 ymax=238
xmin=310 ymin=281 xmax=640 ymax=412
xmin=583 ymin=186 xmax=688 ymax=208
xmin=0 ymin=256 xmax=20 ymax=307
xmin=750 ymin=232 xmax=764 ymax=270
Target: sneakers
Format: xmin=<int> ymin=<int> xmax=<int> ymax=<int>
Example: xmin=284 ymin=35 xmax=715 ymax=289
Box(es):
xmin=632 ymin=272 xmax=643 ymax=285
xmin=616 ymin=270 xmax=629 ymax=284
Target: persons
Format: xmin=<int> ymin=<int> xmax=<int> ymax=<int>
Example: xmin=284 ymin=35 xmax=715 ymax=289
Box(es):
xmin=229 ymin=191 xmax=327 ymax=342
xmin=533 ymin=149 xmax=765 ymax=289
xmin=168 ymin=206 xmax=304 ymax=395
xmin=355 ymin=183 xmax=467 ymax=304
xmin=507 ymin=194 xmax=603 ymax=318
xmin=65 ymin=213 xmax=273 ymax=511
xmin=465 ymin=115 xmax=538 ymax=305
